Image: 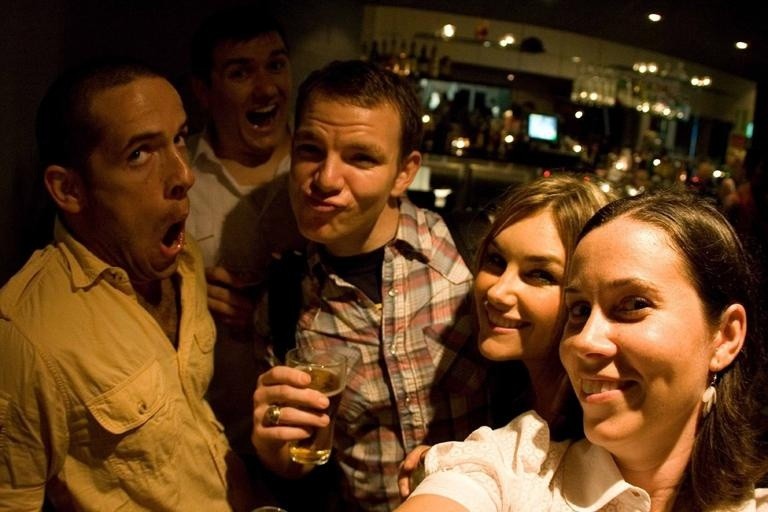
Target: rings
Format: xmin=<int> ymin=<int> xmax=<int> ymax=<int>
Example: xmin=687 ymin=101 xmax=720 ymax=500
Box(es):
xmin=265 ymin=404 xmax=280 ymax=423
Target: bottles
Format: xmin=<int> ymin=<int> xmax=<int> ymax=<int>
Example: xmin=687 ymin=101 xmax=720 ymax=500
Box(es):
xmin=367 ymin=32 xmax=523 ymax=167
xmin=592 ymin=131 xmax=724 ymax=207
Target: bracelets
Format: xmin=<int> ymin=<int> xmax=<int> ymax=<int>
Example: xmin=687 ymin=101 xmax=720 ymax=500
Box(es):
xmin=251 ymin=505 xmax=285 ymax=512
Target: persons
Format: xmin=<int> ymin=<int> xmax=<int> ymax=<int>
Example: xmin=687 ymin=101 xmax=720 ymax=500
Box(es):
xmin=252 ymin=58 xmax=496 ymax=511
xmin=396 ymin=177 xmax=619 ymax=502
xmin=52 ymin=20 xmax=292 ymax=463
xmin=392 ymin=188 xmax=768 ymax=511
xmin=424 ymin=83 xmax=753 ymax=221
xmin=0 ymin=56 xmax=289 ymax=511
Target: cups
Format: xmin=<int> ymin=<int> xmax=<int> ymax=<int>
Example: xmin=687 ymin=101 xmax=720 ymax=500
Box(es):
xmin=281 ymin=346 xmax=348 ymax=466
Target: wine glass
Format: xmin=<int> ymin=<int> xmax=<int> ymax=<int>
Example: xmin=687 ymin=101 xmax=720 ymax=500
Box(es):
xmin=566 ymin=58 xmax=694 ymax=122
